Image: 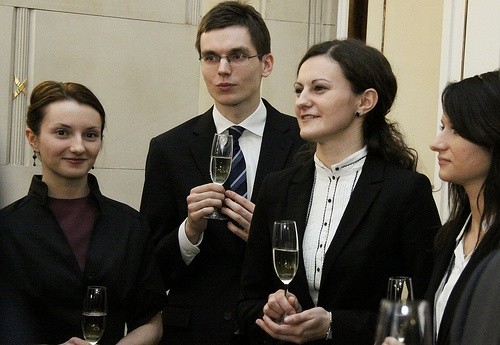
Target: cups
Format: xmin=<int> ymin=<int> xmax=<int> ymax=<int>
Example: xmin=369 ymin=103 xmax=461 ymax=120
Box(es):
xmin=375 ymin=297 xmax=435 ymax=345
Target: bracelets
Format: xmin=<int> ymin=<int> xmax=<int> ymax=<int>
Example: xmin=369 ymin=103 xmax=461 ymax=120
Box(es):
xmin=325 ymin=311 xmax=333 ymax=340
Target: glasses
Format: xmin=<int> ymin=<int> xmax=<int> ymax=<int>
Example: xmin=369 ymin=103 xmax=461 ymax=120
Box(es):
xmin=199 ymin=52 xmax=266 ymax=65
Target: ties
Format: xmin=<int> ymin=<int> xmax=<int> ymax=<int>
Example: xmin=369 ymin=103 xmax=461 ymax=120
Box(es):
xmin=217 ymin=126 xmax=247 ymax=218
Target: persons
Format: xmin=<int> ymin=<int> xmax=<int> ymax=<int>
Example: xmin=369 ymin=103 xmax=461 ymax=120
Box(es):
xmin=239 ymin=40 xmax=443 ymax=345
xmin=381 ymin=70 xmax=500 ymax=344
xmin=0 ymin=81 xmax=169 ymax=345
xmin=140 ymin=1 xmax=307 ymax=345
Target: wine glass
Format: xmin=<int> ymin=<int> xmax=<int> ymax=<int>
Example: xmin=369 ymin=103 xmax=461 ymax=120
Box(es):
xmin=203 ymin=133 xmax=233 ymax=220
xmin=387 ymin=277 xmax=414 ymax=345
xmin=81 ymin=286 xmax=107 ymax=345
xmin=272 ymin=221 xmax=299 ymax=326
